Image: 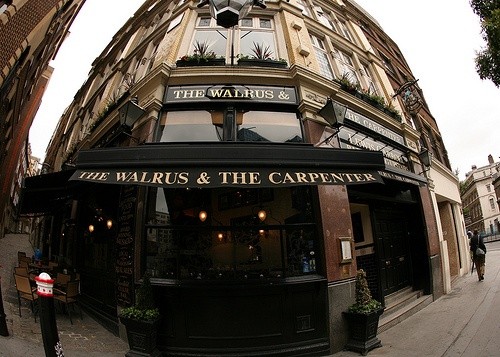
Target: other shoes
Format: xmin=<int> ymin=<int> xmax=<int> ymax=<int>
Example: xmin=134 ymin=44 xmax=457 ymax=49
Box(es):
xmin=478 ymin=274 xmax=484 ymax=281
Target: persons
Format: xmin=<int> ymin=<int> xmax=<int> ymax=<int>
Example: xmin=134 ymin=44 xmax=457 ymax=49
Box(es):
xmin=467 ymin=228 xmax=487 ymax=281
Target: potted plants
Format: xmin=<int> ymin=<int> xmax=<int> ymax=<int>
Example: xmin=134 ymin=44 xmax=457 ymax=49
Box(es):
xmin=362 ymin=84 xmax=385 ymax=111
xmin=333 ymin=70 xmax=361 ymax=99
xmin=176 ymin=40 xmax=226 ymax=66
xmin=237 ymin=41 xmax=287 ymax=68
xmin=342 ymin=269 xmax=387 ymax=356
xmin=384 ymin=101 xmax=401 ymax=122
xmin=118 ymin=273 xmax=164 ymax=357
xmin=72 ymin=88 xmax=130 ymax=164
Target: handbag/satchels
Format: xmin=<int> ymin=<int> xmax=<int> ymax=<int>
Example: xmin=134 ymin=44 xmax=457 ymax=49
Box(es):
xmin=475 ymin=248 xmax=485 ymax=256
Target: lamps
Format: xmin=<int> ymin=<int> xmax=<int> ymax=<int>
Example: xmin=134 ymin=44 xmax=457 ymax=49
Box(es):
xmin=118 ymin=94 xmax=145 ymax=146
xmin=199 ymin=207 xmax=222 ymax=226
xmin=417 ymin=147 xmax=432 ymax=176
xmin=258 ymin=205 xmax=282 ymax=226
xmin=317 ymin=96 xmax=348 ymax=146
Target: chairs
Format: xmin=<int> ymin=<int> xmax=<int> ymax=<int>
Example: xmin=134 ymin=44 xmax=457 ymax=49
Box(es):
xmin=13 ymin=251 xmax=83 ymax=325
